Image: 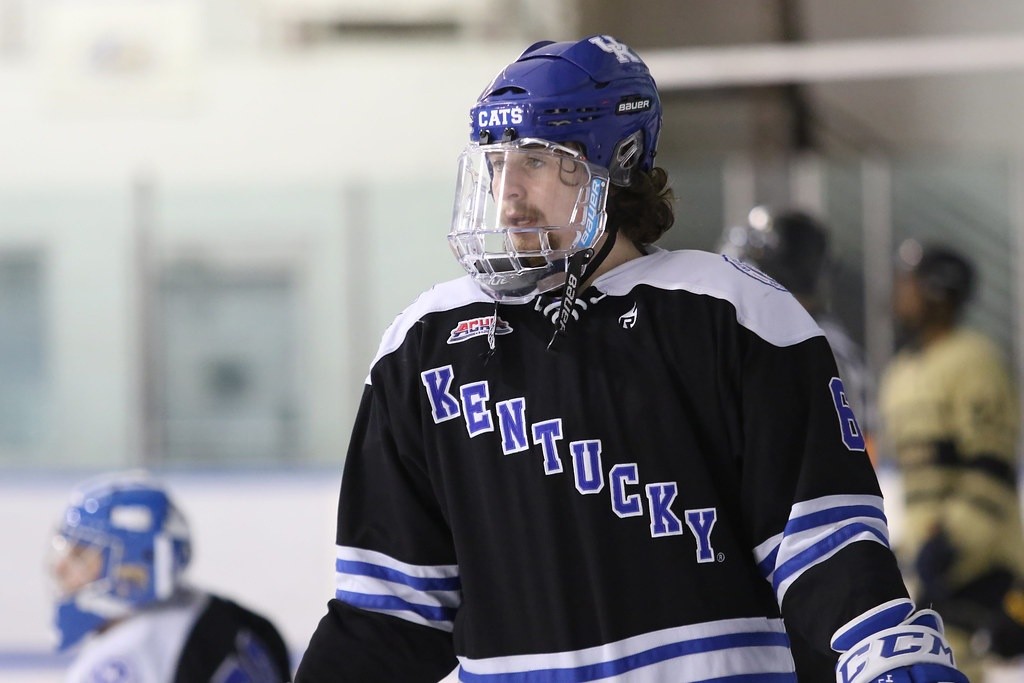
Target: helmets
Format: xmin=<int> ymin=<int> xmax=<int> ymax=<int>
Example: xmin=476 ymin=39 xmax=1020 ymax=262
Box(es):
xmin=53 ymin=482 xmax=187 ymax=649
xmin=907 ymin=248 xmax=978 ymax=308
xmin=447 ymin=33 xmax=661 ymax=301
xmin=739 ymin=208 xmax=824 ymax=287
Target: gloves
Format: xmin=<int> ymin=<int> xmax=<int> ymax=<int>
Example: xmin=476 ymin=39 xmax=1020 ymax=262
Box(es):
xmin=828 ymin=597 xmax=971 ymax=683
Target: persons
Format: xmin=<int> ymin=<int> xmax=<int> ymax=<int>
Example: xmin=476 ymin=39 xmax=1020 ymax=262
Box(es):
xmin=740 ymin=206 xmax=906 ymax=552
xmin=293 ymin=33 xmax=973 ymax=683
xmin=877 ymin=237 xmax=1024 ymax=683
xmin=44 ymin=479 xmax=293 ymax=683
